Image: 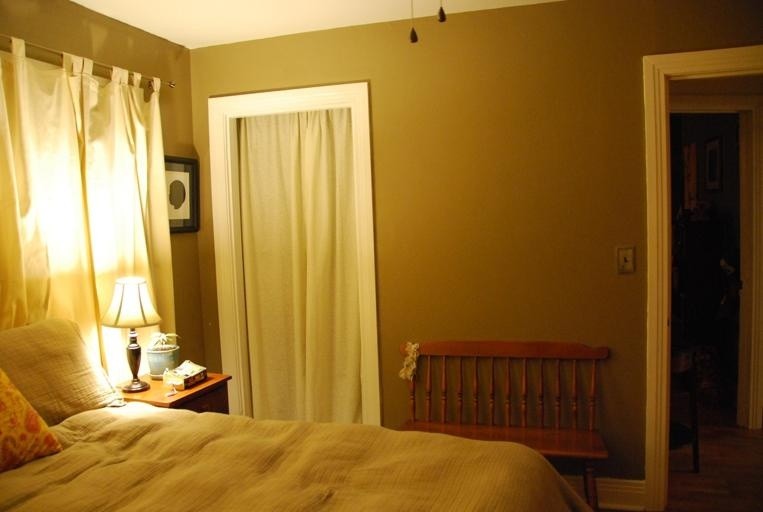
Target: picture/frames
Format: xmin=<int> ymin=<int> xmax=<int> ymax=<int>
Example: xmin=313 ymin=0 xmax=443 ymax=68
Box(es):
xmin=703 ymin=137 xmax=723 ymax=193
xmin=160 ymin=155 xmax=200 ymax=233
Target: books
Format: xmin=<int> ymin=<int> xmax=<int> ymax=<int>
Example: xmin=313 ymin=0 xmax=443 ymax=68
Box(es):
xmin=162 ymin=359 xmax=207 ymax=391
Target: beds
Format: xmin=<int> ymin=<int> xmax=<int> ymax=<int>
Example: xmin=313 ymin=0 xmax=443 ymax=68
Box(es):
xmin=1 ymin=319 xmax=554 ymax=512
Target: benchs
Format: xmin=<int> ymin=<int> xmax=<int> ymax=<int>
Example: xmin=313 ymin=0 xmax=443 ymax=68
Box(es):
xmin=396 ymin=338 xmax=612 ymax=512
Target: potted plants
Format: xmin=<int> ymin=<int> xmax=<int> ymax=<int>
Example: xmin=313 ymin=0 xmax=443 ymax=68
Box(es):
xmin=145 ymin=330 xmax=182 ymax=380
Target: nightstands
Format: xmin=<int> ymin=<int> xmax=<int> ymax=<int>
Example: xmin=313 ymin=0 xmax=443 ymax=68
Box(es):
xmin=110 ymin=368 xmax=232 ymax=417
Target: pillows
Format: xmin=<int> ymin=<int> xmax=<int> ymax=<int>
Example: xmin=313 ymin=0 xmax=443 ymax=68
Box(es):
xmin=0 ymin=321 xmax=120 ymax=474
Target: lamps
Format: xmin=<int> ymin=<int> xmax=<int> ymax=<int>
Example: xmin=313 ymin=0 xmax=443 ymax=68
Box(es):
xmin=99 ymin=276 xmax=163 ymax=394
